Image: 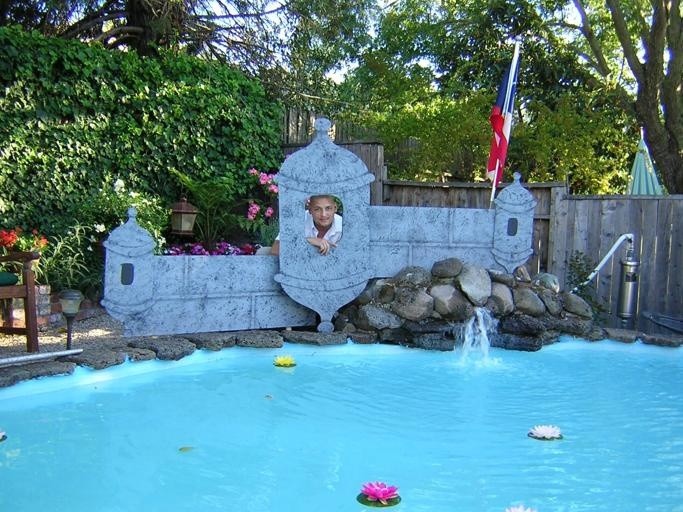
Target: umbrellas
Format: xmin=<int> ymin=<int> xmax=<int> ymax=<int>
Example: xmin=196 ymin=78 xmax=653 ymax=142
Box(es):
xmin=627 ymin=127 xmax=662 ymax=195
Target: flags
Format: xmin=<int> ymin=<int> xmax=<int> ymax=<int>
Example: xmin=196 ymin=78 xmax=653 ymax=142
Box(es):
xmin=486 ymin=52 xmax=520 ymax=187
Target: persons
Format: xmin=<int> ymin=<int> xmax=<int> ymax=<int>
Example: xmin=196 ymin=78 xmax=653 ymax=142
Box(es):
xmin=270 ymin=195 xmax=343 ymax=255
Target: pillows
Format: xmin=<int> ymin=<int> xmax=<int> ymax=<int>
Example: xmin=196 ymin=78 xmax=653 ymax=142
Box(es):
xmin=0 ymin=272 xmax=18 ymax=286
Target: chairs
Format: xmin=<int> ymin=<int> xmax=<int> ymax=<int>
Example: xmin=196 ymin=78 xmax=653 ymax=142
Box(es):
xmin=0 ymin=248 xmax=41 ymax=352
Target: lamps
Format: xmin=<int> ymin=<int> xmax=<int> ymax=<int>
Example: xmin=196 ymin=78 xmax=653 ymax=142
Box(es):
xmin=51 ymin=288 xmax=86 ymax=364
xmin=165 ymin=195 xmax=199 ymax=246
